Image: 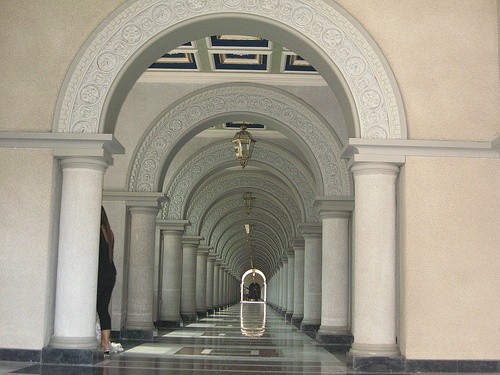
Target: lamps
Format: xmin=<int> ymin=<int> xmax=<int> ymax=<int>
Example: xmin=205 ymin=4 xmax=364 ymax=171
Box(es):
xmin=242 ymin=192 xmax=256 ymax=216
xmin=244 ymin=223 xmax=254 ymax=235
xmin=231 ymin=121 xmax=258 ymax=169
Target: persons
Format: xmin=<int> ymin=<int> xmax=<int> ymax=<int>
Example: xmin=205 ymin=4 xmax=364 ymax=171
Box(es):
xmin=244 ymin=287 xmax=260 ymax=301
xmin=97 ymin=205 xmax=115 ymax=353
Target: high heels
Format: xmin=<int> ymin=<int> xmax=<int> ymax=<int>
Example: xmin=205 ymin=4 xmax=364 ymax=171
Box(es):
xmin=102 ymin=345 xmax=110 ymax=355
